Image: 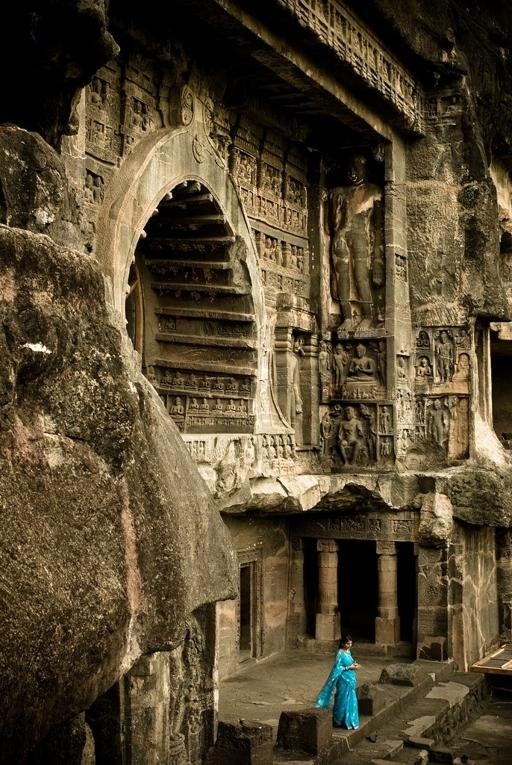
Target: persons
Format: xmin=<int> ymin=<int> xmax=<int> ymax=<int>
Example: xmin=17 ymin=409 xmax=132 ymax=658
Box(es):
xmin=456 ymin=330 xmax=471 ymax=350
xmin=416 ymin=331 xmax=430 ymax=347
xmin=313 ymin=339 xmax=387 ymax=389
xmin=320 ymin=415 xmax=330 ymax=455
xmin=380 ymin=406 xmax=390 ymax=435
xmin=322 ymin=146 xmax=380 ymax=333
xmin=435 ymin=331 xmax=455 ymax=382
xmin=336 ymin=406 xmax=365 ymax=465
xmin=427 ymin=398 xmax=446 ymax=447
xmin=312 ymin=633 xmax=364 ymax=731
xmin=380 ymin=438 xmax=392 ymax=459
xmin=417 ymin=358 xmax=431 ymax=378
